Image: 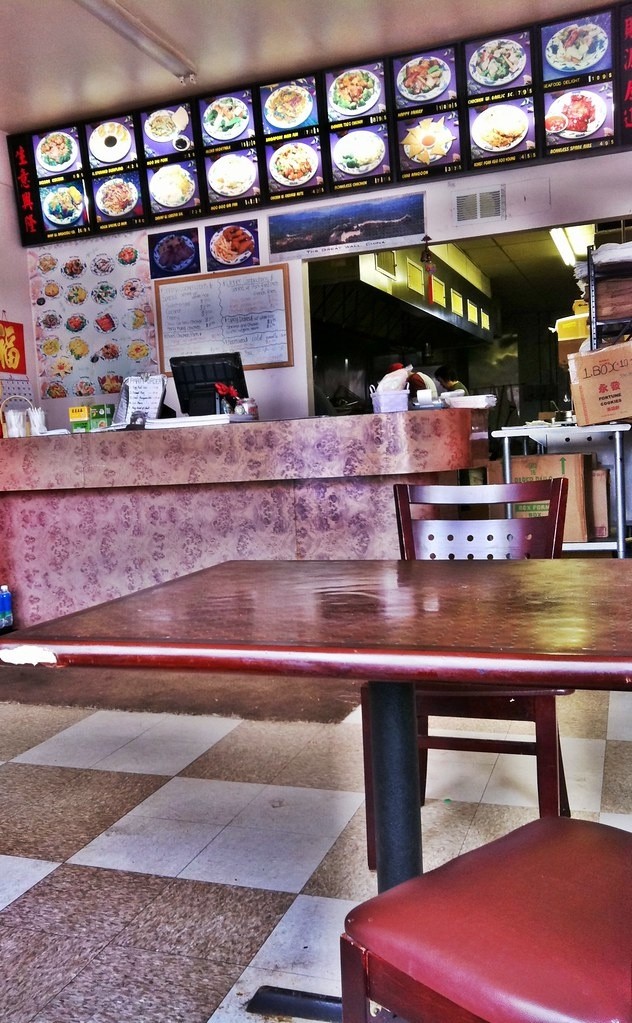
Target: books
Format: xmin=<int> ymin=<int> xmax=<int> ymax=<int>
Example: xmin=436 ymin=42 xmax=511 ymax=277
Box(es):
xmin=144 ymin=414 xmax=253 ymax=430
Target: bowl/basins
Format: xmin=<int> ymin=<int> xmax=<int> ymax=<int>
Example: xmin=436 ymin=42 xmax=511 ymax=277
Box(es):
xmin=441 ymin=389 xmax=465 ymax=399
xmin=370 ymin=389 xmax=410 ymax=412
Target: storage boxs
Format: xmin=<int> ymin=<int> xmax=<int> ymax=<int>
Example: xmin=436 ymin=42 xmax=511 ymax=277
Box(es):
xmin=486 ymin=450 xmax=610 ymax=543
xmin=552 ymin=279 xmax=632 ymax=426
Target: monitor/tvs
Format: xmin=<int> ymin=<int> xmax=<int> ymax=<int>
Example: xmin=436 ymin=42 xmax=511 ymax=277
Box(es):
xmin=169 ymin=352 xmax=250 ymax=416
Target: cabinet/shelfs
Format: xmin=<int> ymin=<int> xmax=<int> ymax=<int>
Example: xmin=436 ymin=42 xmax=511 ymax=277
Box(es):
xmin=586 ymin=241 xmax=632 ymax=425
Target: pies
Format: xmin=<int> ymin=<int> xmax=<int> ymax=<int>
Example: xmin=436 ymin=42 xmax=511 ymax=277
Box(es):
xmin=400 ymin=116 xmax=457 ymax=166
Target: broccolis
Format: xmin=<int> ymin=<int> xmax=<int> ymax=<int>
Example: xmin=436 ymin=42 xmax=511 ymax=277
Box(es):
xmin=209 ymin=98 xmax=247 ymax=132
xmin=333 ymin=71 xmax=375 ymax=110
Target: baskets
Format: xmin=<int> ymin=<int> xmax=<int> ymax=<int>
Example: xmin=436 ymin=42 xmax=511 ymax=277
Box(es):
xmin=0 ymin=396 xmax=33 ymax=438
xmin=369 ymin=382 xmax=410 ymax=413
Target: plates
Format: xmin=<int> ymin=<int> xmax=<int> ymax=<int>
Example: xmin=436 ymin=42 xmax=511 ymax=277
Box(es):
xmin=210 ymin=226 xmax=255 ymax=265
xmin=471 ymin=104 xmax=528 ymax=152
xmin=36 ymin=70 xmax=385 ymax=225
xmin=396 ymin=56 xmax=451 ymax=101
xmin=469 ymin=39 xmax=527 ymax=86
xmin=154 ymin=235 xmax=196 ymax=272
xmin=545 ymin=24 xmax=608 ymax=72
xmin=404 ymin=122 xmax=452 ymax=163
xmin=547 ymin=90 xmax=606 ymax=139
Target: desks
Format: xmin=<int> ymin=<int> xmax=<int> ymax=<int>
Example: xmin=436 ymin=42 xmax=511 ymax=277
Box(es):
xmin=491 ymin=422 xmax=631 ymax=559
xmin=0 ymin=558 xmax=632 ymax=1023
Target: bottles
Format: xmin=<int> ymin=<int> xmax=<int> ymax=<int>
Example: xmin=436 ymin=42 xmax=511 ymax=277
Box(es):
xmin=0 ymin=585 xmax=13 ymax=629
xmin=400 ymin=343 xmax=432 ymax=366
xmin=221 ymin=397 xmax=259 ymax=420
xmin=561 ymin=394 xmax=571 ymax=411
xmin=376 ymin=364 xmax=414 ymax=391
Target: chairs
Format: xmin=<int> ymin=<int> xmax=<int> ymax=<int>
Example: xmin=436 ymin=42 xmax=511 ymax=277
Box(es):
xmin=338 ymin=815 xmax=632 ymax=1023
xmin=361 ymin=477 xmax=569 ymax=876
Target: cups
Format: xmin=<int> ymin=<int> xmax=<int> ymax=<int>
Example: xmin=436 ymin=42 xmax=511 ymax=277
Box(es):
xmin=4 ymin=412 xmax=26 ymax=437
xmin=417 ymin=388 xmax=432 ymax=405
xmin=29 ymin=412 xmax=47 ymax=436
xmin=555 ymin=411 xmax=566 ymax=422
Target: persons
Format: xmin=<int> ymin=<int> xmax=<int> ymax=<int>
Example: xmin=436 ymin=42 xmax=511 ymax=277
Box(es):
xmin=434 ymin=366 xmax=469 ymax=396
xmin=387 ymin=363 xmax=438 ymax=398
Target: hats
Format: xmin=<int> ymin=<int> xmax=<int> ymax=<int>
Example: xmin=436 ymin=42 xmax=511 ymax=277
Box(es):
xmin=385 ymin=363 xmax=403 ymax=372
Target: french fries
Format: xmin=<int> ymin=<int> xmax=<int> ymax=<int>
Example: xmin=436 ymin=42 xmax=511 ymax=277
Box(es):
xmin=213 ymin=235 xmax=237 ymax=262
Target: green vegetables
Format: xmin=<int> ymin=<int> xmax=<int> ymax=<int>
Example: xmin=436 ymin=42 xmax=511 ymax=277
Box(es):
xmin=43 ymin=137 xmax=73 ymax=165
xmin=477 ymin=49 xmax=523 ymax=81
